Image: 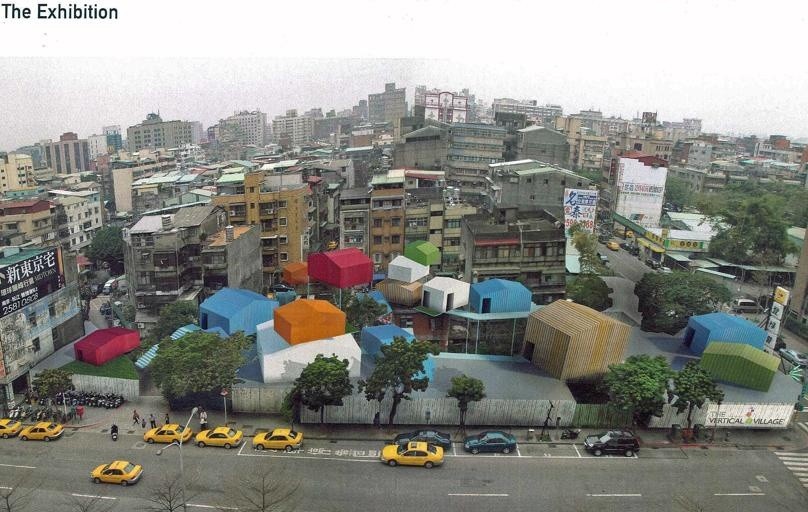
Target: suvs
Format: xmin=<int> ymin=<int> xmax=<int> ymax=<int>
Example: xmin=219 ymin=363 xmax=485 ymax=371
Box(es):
xmin=595 ymin=252 xmax=610 ymax=264
xmin=583 ymin=427 xmax=641 ymax=457
xmin=758 ymin=293 xmax=774 ymax=310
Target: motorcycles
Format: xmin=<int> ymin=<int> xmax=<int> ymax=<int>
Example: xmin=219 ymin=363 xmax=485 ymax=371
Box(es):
xmin=7 ymin=385 xmax=128 ymax=423
xmin=112 ymin=431 xmax=118 ymax=442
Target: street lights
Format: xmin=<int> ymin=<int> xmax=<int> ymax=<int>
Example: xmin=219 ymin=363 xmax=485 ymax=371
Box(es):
xmin=509 ymin=219 xmax=525 ymax=280
xmin=155 ymin=406 xmax=200 ymax=511
xmin=779 ymin=352 xmax=786 ymax=375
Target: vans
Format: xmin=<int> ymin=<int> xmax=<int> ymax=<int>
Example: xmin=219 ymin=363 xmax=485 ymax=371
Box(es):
xmin=732 ymin=299 xmax=763 ymax=313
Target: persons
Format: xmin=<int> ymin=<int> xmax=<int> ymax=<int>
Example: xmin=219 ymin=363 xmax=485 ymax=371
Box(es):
xmin=373 ymin=411 xmax=380 ymax=425
xmin=164 ymin=413 xmax=170 ymax=423
xmin=141 ymin=419 xmax=147 ymax=429
xmin=199 ymin=409 xmax=208 ymax=430
xmin=110 ymin=424 xmax=118 ymax=434
xmin=132 ymin=410 xmax=140 ymax=425
xmin=149 ymin=413 xmax=157 ymax=429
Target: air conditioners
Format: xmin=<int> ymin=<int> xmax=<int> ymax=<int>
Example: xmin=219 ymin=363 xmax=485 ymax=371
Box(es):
xmin=138 ymin=323 xmax=144 ymax=329
xmin=268 ymin=209 xmax=274 ymax=214
xmin=230 ymin=210 xmax=235 ymax=217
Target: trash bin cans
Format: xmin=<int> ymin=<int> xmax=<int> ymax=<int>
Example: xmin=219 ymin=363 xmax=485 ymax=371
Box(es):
xmin=527 ymin=429 xmax=535 ymax=440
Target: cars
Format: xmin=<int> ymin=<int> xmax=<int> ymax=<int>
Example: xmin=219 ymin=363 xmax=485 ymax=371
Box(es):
xmin=268 ymin=282 xmax=296 ymax=292
xmin=18 ymin=420 xmax=65 ymax=442
xmin=327 ymin=240 xmax=339 ymax=250
xmin=777 ymin=348 xmax=808 ymax=368
xmin=597 ymin=230 xmax=634 ymax=253
xmin=252 ymin=427 xmax=304 ymax=453
xmin=644 ymin=257 xmax=661 ymax=269
xmin=656 ymin=267 xmax=672 ymax=274
xmin=463 ymin=430 xmax=518 ymax=455
xmin=379 ymin=441 xmax=446 ymax=469
xmin=0 ymin=418 xmax=22 ymax=439
xmin=89 ymin=459 xmax=144 ymax=486
xmin=392 ymin=428 xmax=451 ymax=450
xmin=195 ymin=426 xmax=244 ymax=449
xmin=774 ymin=336 xmax=787 ymax=351
xmin=143 ymin=423 xmax=193 ymax=444
xmin=80 ymin=278 xmax=130 ymax=327
